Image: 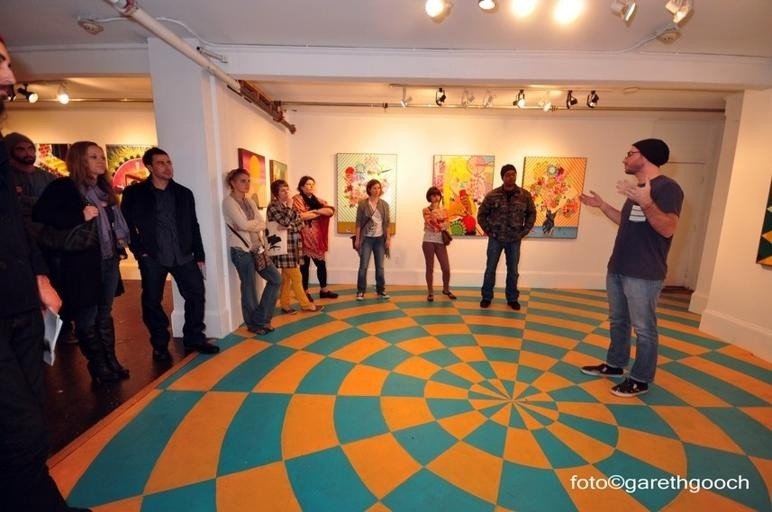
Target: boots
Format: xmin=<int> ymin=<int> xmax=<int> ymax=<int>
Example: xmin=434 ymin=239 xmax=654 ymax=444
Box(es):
xmin=75 ymin=311 xmax=121 ymax=386
xmin=92 ymin=316 xmax=130 ymax=377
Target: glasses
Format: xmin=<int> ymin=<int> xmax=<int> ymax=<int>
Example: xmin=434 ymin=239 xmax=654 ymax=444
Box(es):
xmin=627 ymin=151 xmax=639 ymax=157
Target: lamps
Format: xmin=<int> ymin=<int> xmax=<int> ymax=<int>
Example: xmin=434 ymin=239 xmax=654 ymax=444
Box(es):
xmin=8 ymin=79 xmax=71 ymax=105
xmin=424 ymin=0 xmax=453 ymax=18
xmin=388 ymin=83 xmax=617 ymax=112
xmin=477 ymin=0 xmax=497 ymax=10
xmin=662 ymin=0 xmax=698 ymax=25
xmin=609 ymin=0 xmax=635 ymax=22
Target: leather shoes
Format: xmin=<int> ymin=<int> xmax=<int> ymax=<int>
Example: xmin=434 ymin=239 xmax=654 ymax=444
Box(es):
xmin=151 ymin=347 xmax=174 ymax=365
xmin=182 ymin=338 xmax=220 ymax=355
xmin=62 ymin=333 xmax=80 ymax=345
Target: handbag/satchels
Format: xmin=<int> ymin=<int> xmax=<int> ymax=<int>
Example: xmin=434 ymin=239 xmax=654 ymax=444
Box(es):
xmin=350 ymin=234 xmax=359 ymax=252
xmin=251 ymin=249 xmax=274 ymax=272
xmin=262 ymin=220 xmax=289 ymax=257
xmin=32 ymin=218 xmax=104 ymax=286
xmin=441 ymin=229 xmax=453 ymax=246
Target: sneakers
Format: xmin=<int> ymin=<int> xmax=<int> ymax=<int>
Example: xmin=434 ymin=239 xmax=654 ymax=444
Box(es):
xmin=377 ymin=291 xmax=392 ymax=299
xmin=249 ymin=322 xmax=276 ymax=336
xmin=507 ymin=301 xmax=521 ymax=310
xmin=281 ymin=307 xmax=298 ymax=315
xmin=609 ymin=376 xmax=650 ymax=399
xmin=305 ymin=294 xmax=313 ymax=302
xmin=480 ymin=299 xmax=492 ymax=308
xmin=319 ymin=290 xmax=338 ymax=299
xmin=302 ymin=305 xmax=325 ymax=313
xmin=579 ymin=362 xmax=625 ymax=379
xmin=427 ymin=294 xmax=434 ymax=301
xmin=442 ymin=289 xmax=457 ymax=299
xmin=356 ymin=292 xmax=364 ymax=301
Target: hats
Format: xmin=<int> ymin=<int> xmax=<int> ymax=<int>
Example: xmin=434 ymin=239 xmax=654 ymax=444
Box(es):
xmin=632 ymin=138 xmax=669 ymax=168
xmin=4 ymin=132 xmax=36 ymax=157
xmin=501 ymin=164 xmax=517 ymax=180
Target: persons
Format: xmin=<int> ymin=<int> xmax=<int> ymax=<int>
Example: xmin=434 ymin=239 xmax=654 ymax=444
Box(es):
xmin=476 ymin=163 xmax=537 ymax=311
xmin=264 ymin=179 xmax=325 ymax=314
xmin=353 ymin=179 xmax=391 ymax=301
xmin=291 ymin=174 xmax=339 ymax=302
xmin=0 ymin=35 xmax=132 ymax=512
xmin=221 ymin=168 xmax=283 ymax=336
xmin=578 ymin=138 xmax=685 ymax=396
xmin=420 ymin=186 xmax=457 ymax=302
xmin=120 ymin=146 xmax=220 ymax=364
